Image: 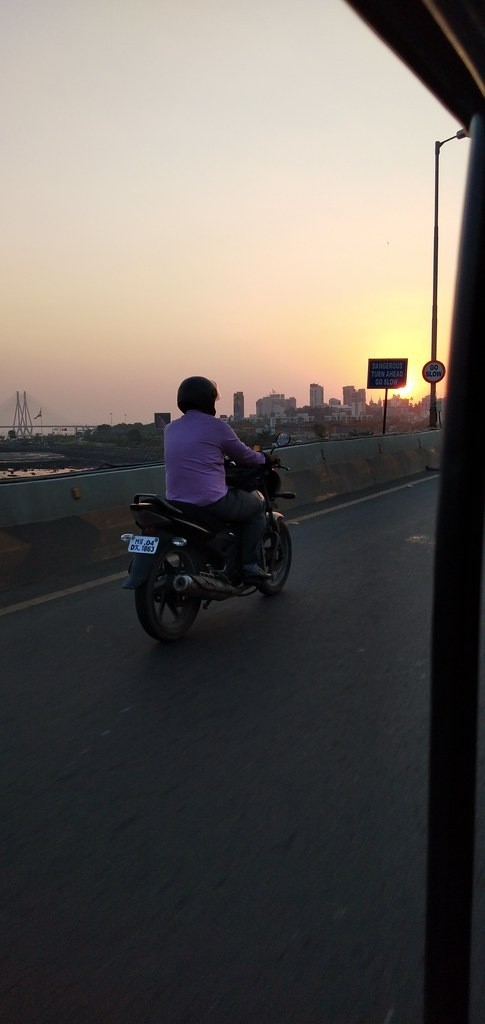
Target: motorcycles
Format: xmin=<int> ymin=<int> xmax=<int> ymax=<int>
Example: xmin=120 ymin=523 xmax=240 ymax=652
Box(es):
xmin=120 ymin=432 xmax=297 ymax=643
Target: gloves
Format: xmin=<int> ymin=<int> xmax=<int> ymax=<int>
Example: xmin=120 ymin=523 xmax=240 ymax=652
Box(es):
xmin=262 ymin=451 xmax=281 ymax=467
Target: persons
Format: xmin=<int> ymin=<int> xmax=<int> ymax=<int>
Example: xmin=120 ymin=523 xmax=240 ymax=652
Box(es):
xmin=163 ymin=376 xmax=282 ymax=580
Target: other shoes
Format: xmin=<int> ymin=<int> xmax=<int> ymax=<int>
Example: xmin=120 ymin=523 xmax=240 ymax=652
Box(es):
xmin=237 ymin=564 xmax=271 ymax=582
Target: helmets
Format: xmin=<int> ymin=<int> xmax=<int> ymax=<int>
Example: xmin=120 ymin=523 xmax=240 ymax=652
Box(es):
xmin=177 ymin=376 xmax=217 ymax=416
